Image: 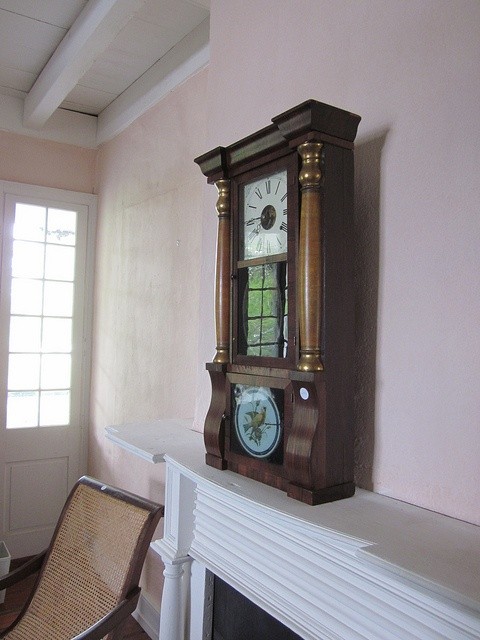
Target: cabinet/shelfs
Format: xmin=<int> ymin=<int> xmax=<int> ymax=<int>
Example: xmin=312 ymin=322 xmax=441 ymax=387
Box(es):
xmin=101 ymin=420 xmax=205 ymax=463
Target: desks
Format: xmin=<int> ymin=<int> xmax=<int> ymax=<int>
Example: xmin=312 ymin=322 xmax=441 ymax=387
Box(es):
xmin=149 ymin=443 xmax=478 ymax=639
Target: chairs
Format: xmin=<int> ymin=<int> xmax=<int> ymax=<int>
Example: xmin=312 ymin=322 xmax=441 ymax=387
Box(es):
xmin=3 ymin=476 xmax=164 ymax=640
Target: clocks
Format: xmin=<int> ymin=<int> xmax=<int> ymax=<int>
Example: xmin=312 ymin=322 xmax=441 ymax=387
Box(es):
xmin=194 ymin=99 xmax=362 ymax=507
